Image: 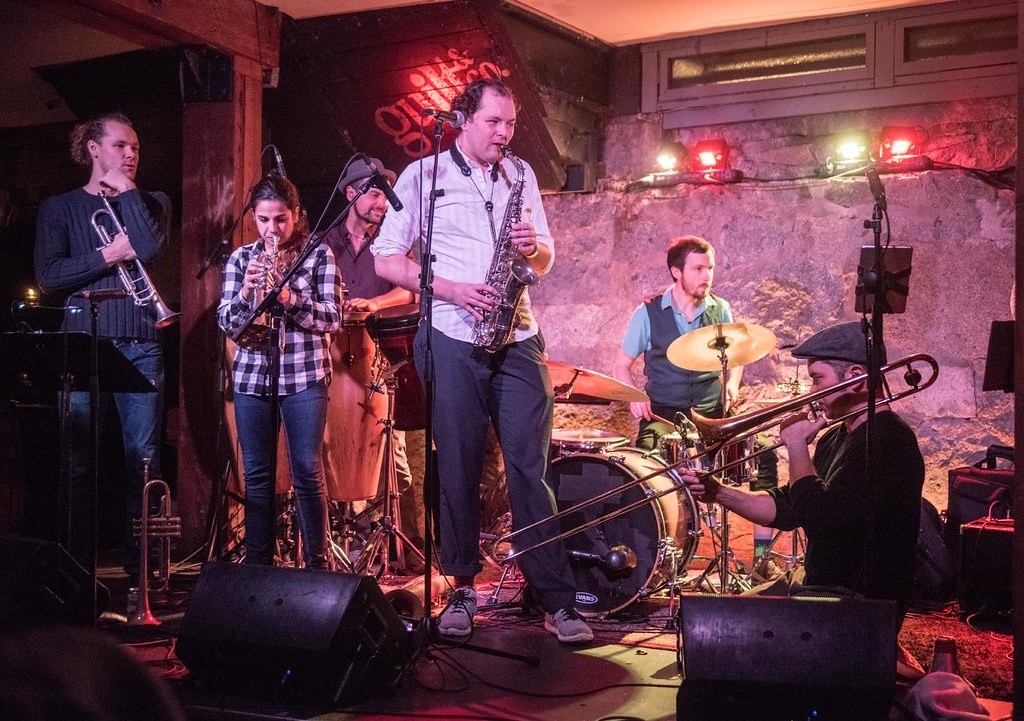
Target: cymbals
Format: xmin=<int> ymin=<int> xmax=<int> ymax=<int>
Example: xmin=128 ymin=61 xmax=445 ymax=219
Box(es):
xmin=666 ymin=321 xmax=778 ymax=373
xmin=545 ymin=359 xmax=651 ymax=408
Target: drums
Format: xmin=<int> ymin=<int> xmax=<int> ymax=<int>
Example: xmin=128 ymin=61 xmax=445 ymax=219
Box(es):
xmin=659 ymin=428 xmax=762 ymax=484
xmin=548 ymin=427 xmax=630 ymax=451
xmin=214 ymin=310 xmax=394 ymax=502
xmin=366 ymin=301 xmax=435 ymax=432
xmin=546 ymin=446 xmax=702 ymax=621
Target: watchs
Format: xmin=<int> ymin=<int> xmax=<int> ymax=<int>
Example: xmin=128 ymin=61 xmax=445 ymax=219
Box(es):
xmin=522 ymin=240 xmax=542 ymax=259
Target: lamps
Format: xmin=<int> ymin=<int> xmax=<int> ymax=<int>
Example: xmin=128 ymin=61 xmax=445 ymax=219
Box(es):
xmin=879 ymin=125 xmax=921 ymax=161
xmin=655 ymin=141 xmax=689 ymax=169
xmin=693 ymin=139 xmax=730 ymax=171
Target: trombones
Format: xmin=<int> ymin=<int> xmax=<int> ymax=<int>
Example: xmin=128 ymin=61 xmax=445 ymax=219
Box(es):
xmin=492 ymin=352 xmax=940 ymax=566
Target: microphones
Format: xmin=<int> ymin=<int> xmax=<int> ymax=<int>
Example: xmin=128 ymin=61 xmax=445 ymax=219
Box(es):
xmin=423 ymin=107 xmax=465 ymax=127
xmin=364 ymin=155 xmax=404 ymax=211
xmin=868 ymin=168 xmax=887 ymax=210
xmin=275 ymin=148 xmax=288 ymax=179
xmin=72 ymin=287 xmax=129 ymax=298
xmin=676 ymin=411 xmax=697 ymax=432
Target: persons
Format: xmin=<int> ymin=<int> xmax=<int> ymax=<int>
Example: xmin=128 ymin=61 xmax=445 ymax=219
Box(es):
xmin=613 ymin=235 xmax=746 ymax=492
xmin=677 ymin=320 xmax=926 ymax=721
xmin=315 ymin=157 xmax=434 ymax=572
xmin=368 ymin=77 xmax=599 ymax=644
xmin=31 ymin=113 xmax=174 ymax=610
xmin=0 ymin=617 xmax=188 ymax=721
xmin=216 ymin=174 xmax=343 ymax=575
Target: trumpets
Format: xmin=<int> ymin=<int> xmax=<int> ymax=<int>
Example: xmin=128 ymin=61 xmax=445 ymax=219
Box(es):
xmin=122 ymin=456 xmax=182 ymax=628
xmin=90 ymin=188 xmax=184 ymax=329
xmin=238 ymin=234 xmax=284 ymax=353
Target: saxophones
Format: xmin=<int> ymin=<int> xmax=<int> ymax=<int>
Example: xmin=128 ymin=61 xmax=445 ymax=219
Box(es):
xmin=472 ymin=147 xmax=540 ymax=350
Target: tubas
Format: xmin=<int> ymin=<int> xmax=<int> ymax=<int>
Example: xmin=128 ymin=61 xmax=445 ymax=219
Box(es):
xmin=384 ymin=566 xmax=457 ymax=625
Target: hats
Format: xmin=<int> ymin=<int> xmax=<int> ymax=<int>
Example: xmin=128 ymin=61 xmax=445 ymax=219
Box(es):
xmin=337 ymin=158 xmax=397 ymax=194
xmin=791 ymin=320 xmax=887 ymax=369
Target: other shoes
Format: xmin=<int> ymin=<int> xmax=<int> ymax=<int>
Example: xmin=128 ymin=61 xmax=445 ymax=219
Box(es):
xmin=125 ymin=584 xmax=188 ymax=613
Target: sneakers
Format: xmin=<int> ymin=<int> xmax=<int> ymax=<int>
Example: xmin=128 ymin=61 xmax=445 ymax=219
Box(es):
xmin=751 ymin=557 xmax=786 ymax=586
xmin=437 ymin=588 xmax=477 ymax=635
xmin=544 ymin=606 xmax=596 ymax=642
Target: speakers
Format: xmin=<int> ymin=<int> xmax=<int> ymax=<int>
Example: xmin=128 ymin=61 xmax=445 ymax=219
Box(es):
xmin=0 ymin=534 xmax=111 ymax=634
xmin=179 ymin=560 xmax=411 ymax=705
xmin=677 ymin=594 xmax=899 ymax=720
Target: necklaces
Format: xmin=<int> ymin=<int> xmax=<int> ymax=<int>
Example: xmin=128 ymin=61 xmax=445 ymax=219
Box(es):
xmin=341 ymin=222 xmax=376 ymax=243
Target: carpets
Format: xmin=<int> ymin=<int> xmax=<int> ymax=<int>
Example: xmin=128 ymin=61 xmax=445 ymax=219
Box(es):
xmin=471 ymin=578 xmax=731 ymax=653
